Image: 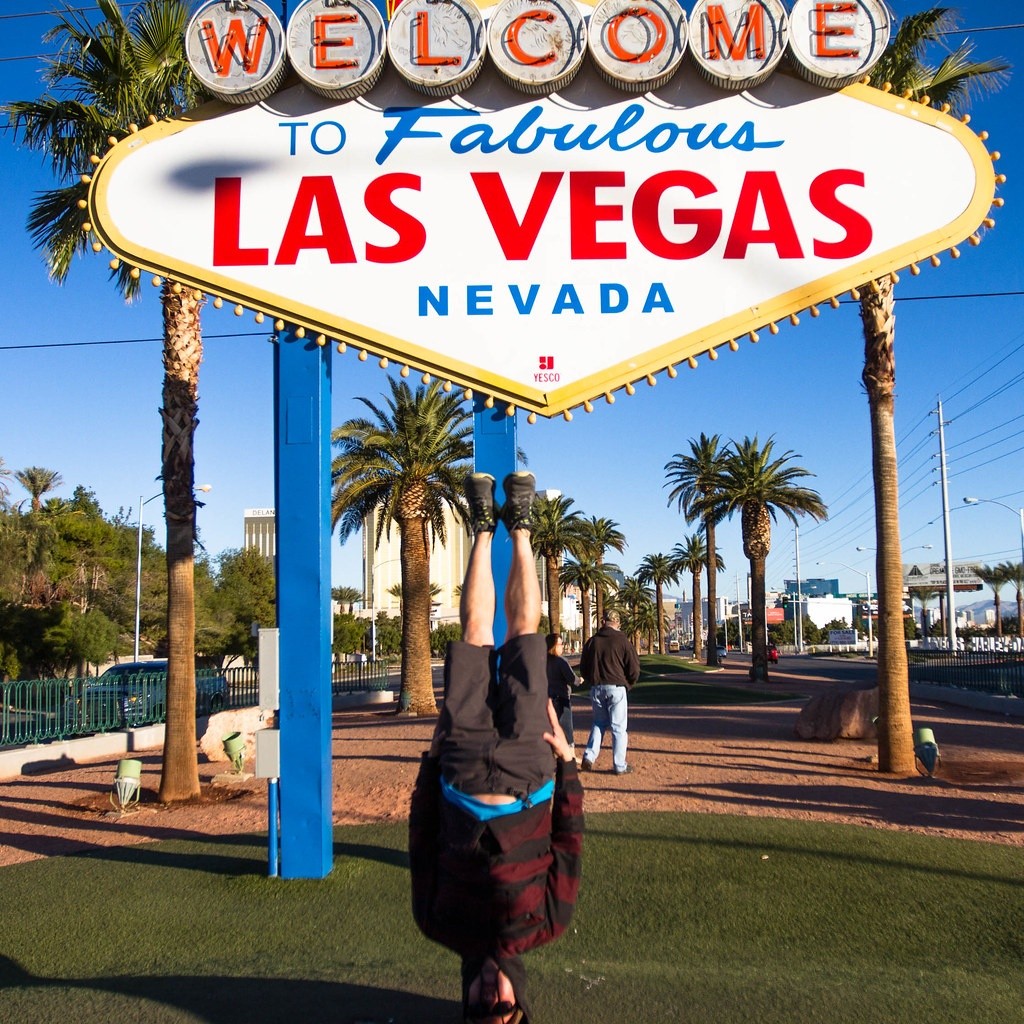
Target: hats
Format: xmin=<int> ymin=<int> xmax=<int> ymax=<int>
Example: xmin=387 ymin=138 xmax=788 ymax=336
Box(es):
xmin=601 ymin=609 xmax=620 ymax=622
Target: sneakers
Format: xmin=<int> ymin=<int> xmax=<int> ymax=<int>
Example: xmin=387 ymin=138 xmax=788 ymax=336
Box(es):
xmin=501 ymin=472 xmax=535 ymax=537
xmin=463 ymin=472 xmax=500 ymax=540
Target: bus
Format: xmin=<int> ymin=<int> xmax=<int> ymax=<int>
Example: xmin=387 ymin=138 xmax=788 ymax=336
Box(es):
xmin=669 ymin=641 xmax=679 ymax=653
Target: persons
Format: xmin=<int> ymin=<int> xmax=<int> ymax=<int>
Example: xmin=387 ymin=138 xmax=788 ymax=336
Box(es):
xmin=545 ymin=633 xmax=584 ymax=765
xmin=407 ymin=472 xmax=586 ymax=1024
xmin=578 ymin=608 xmax=640 ymax=774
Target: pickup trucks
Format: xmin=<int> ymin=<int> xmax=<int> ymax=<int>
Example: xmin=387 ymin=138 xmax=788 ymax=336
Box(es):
xmin=64 ymin=661 xmax=231 ymax=738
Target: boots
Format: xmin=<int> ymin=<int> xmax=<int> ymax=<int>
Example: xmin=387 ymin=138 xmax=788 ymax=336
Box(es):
xmin=568 ymin=743 xmax=582 ymax=765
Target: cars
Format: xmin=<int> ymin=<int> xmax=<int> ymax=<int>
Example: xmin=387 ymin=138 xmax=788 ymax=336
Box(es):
xmin=765 ymin=645 xmax=778 ymax=664
xmin=653 ymin=643 xmax=686 ymax=651
xmin=716 ymin=646 xmax=727 ymax=658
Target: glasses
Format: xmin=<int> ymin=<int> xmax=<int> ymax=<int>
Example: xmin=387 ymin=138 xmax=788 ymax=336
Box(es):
xmin=557 ymin=642 xmax=564 ymax=646
xmin=468 ymin=1000 xmax=518 ymax=1021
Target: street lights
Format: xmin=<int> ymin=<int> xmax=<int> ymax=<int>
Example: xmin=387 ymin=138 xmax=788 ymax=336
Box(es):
xmin=771 ymin=587 xmax=798 ymax=656
xmin=748 ymin=599 xmax=768 ymax=645
xmin=134 ymin=484 xmax=212 ymax=662
xmin=816 ymin=562 xmax=873 ymax=657
xmin=964 ymin=498 xmax=1024 ymax=568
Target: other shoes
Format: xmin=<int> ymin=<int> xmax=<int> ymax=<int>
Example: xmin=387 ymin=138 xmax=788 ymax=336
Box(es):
xmin=615 ymin=764 xmax=635 ymax=775
xmin=581 ymin=757 xmax=592 ymax=772
